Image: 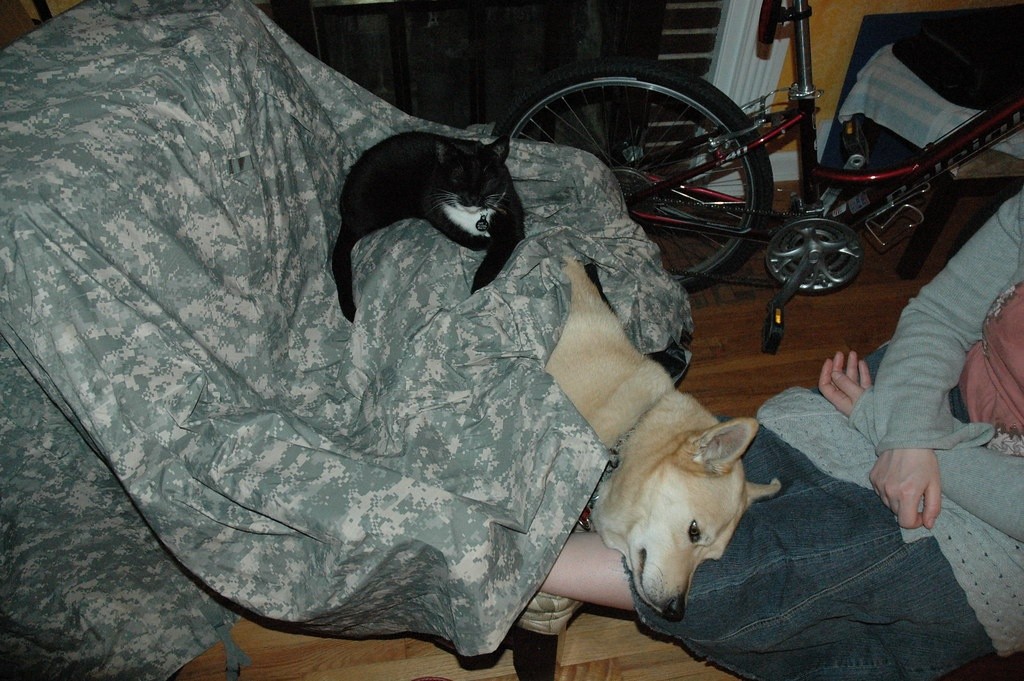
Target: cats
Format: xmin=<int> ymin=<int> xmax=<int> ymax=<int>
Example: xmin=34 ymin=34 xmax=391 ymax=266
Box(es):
xmin=332 ymin=132 xmax=525 ymax=324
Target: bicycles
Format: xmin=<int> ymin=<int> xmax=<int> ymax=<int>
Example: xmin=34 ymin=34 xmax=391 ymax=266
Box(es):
xmin=489 ymin=0 xmax=1024 ymax=359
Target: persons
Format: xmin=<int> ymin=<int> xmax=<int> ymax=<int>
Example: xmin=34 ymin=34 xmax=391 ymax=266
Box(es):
xmin=537 ymin=180 xmax=1024 ymax=681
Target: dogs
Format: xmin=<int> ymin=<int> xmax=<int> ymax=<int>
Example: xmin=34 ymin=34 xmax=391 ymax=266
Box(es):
xmin=545 ymin=255 xmax=779 ymax=622
